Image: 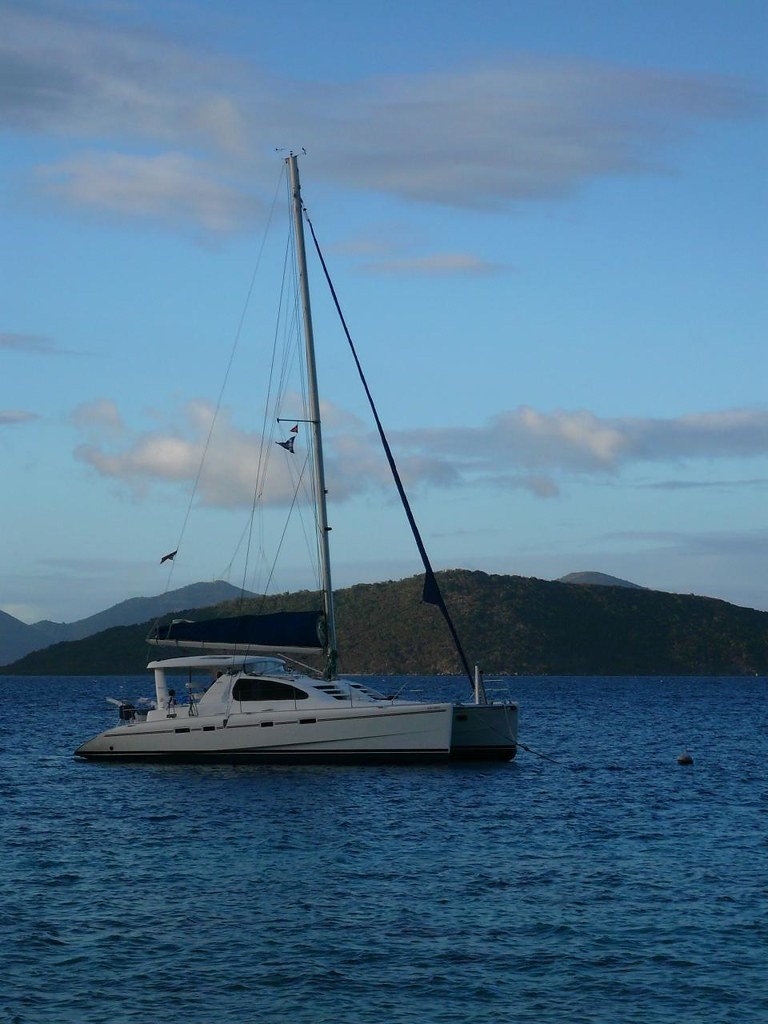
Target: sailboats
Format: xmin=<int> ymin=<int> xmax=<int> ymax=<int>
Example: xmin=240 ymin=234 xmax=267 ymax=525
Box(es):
xmin=73 ymin=150 xmax=519 ymax=767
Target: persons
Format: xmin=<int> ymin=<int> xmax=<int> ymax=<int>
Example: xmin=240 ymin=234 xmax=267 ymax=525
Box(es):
xmin=168 ymin=689 xmax=177 ymax=705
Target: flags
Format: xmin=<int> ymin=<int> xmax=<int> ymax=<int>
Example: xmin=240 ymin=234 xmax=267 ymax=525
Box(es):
xmin=160 ymin=551 xmax=178 ymax=564
xmin=289 ymin=425 xmax=298 ymax=433
xmin=275 ymin=436 xmax=295 ymax=454
